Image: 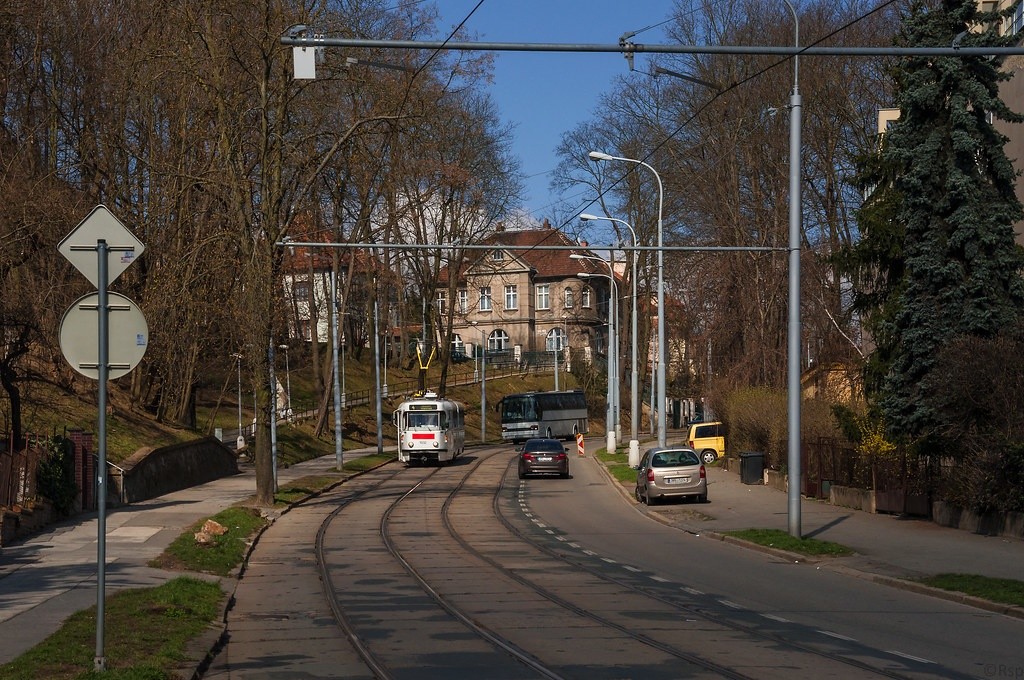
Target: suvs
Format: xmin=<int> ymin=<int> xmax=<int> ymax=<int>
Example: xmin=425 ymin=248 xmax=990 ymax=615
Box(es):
xmin=635 ymin=443 xmax=708 ymax=506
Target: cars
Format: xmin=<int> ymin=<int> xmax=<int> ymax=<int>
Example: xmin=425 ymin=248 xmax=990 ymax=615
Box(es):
xmin=515 ymin=439 xmax=570 ymax=480
xmin=687 ymin=413 xmax=704 ymax=430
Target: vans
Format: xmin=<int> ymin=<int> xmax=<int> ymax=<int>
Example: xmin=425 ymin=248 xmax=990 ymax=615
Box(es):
xmin=684 ymin=422 xmax=726 ymax=464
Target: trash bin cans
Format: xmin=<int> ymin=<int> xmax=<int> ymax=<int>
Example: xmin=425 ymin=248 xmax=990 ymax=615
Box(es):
xmin=738 ymin=450 xmax=765 ymax=486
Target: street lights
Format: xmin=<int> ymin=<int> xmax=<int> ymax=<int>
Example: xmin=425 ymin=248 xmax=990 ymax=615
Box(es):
xmin=580 ymin=213 xmax=643 ymax=471
xmin=590 ymin=152 xmax=666 ymax=449
xmin=280 ymin=345 xmax=293 ymax=416
xmin=570 ymin=254 xmax=622 ymax=455
xmin=472 ymin=322 xmax=479 ymax=383
xmin=562 ymin=316 xmax=567 ymax=345
xmin=233 ymin=353 xmax=245 ymax=450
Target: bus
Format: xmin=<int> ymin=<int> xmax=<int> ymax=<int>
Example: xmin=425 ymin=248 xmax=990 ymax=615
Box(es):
xmin=496 ymin=388 xmax=588 ymax=445
xmin=392 ymin=339 xmax=466 ymax=466
